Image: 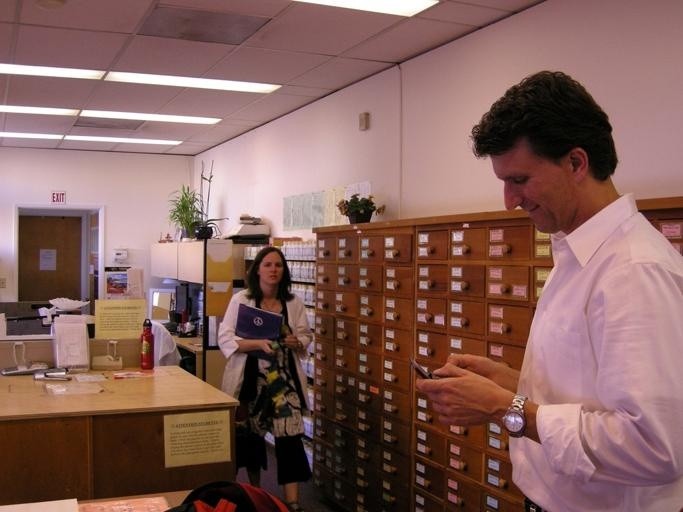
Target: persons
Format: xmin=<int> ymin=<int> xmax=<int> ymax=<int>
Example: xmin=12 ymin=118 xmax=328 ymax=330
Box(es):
xmin=215 ymin=245 xmax=315 ymax=512
xmin=414 ymin=69 xmax=682 ymax=512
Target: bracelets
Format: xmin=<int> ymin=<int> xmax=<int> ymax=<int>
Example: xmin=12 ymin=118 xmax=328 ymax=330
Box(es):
xmin=296 ymin=338 xmax=303 ymax=349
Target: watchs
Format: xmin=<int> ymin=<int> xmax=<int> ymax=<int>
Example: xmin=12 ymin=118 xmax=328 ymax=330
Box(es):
xmin=502 ymin=393 xmax=529 ymax=439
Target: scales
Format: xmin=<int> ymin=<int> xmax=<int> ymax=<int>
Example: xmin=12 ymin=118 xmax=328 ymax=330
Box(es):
xmin=223 ymin=223 xmax=270 ymax=239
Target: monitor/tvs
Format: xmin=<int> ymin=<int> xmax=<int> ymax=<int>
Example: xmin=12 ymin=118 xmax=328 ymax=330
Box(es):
xmin=147 ymin=288 xmax=176 ymax=324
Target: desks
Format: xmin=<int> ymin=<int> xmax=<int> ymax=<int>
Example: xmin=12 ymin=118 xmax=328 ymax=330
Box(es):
xmin=0 ymin=365 xmax=240 ymax=511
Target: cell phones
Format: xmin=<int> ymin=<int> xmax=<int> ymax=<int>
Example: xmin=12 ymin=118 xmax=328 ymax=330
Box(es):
xmin=409 ymin=358 xmax=430 ymax=379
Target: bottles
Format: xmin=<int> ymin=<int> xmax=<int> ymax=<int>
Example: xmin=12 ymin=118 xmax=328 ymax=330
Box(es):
xmin=138 ymin=319 xmax=154 ymax=369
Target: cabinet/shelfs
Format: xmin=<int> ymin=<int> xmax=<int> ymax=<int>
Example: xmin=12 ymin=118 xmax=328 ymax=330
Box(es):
xmin=147 ymin=238 xmax=267 ymax=390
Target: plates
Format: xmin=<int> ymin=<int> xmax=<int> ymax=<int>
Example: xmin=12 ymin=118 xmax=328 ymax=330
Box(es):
xmin=48 ymin=297 xmax=90 ymax=310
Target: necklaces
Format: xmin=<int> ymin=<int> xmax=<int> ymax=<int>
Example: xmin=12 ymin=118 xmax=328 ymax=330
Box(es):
xmin=264 ymin=299 xmax=276 ymax=312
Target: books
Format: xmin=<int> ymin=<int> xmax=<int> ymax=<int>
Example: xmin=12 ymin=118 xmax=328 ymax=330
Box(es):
xmin=52 ymin=315 xmax=89 ymax=368
xmin=234 ymin=303 xmax=284 ymax=362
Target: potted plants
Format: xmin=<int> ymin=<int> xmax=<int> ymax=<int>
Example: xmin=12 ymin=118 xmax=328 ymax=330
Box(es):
xmin=166 ymin=185 xmax=228 ymax=241
